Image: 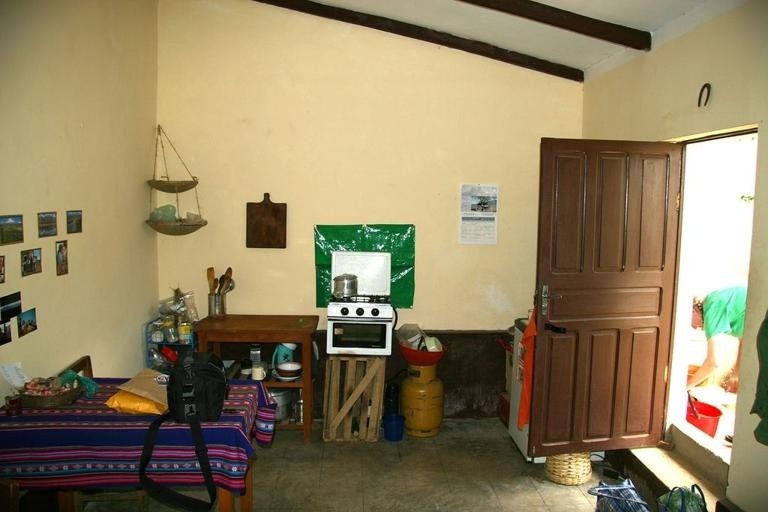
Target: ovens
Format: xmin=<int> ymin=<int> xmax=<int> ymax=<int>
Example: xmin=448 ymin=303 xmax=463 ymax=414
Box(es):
xmin=327 ymin=316 xmax=393 ymax=357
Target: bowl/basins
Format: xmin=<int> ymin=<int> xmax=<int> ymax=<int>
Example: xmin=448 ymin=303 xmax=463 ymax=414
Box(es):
xmin=688 ymin=364 xmax=706 ymax=387
xmin=396 ymin=339 xmax=448 ymax=366
xmin=147 ymin=179 xmax=198 ymax=193
xmin=145 ymin=220 xmax=208 ymax=236
xmin=687 ymin=401 xmax=723 ymax=437
xmin=277 ymin=362 xmax=303 ymax=376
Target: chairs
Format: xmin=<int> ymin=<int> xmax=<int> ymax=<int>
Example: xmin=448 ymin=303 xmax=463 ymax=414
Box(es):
xmin=59 ymin=355 xmax=148 ymax=512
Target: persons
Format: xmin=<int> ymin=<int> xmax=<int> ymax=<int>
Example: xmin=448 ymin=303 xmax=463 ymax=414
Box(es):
xmin=686 ymin=285 xmax=747 ymax=443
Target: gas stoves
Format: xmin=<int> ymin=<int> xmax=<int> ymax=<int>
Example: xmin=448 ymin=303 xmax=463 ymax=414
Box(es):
xmin=325 ymin=294 xmax=393 ymax=319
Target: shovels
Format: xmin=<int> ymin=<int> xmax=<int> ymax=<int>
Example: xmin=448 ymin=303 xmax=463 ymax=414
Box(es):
xmin=207 ymin=267 xmax=232 ymax=295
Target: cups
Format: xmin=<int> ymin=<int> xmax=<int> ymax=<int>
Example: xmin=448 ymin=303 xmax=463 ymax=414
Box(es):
xmin=207 ymin=293 xmax=226 ymax=319
xmin=251 ymin=366 xmax=267 ymax=381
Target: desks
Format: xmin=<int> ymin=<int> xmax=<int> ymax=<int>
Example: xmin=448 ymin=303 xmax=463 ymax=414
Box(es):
xmin=0 ymin=360 xmax=278 ymax=512
xmin=197 ymin=313 xmax=319 ymax=444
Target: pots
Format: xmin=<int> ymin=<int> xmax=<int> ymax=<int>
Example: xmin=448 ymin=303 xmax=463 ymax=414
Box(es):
xmin=269 ymin=390 xmax=291 ymax=421
xmin=333 ymin=273 xmax=358 ymax=299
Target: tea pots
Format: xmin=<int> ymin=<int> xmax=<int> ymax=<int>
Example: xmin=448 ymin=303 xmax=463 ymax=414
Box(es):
xmin=244 ymin=193 xmax=288 ymax=249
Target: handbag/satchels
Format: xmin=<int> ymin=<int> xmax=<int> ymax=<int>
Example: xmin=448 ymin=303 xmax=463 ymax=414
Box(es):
xmin=588 ymin=479 xmax=654 ymax=512
xmin=166 ymin=349 xmax=228 ymax=422
xmin=657 ymin=483 xmax=709 ymax=511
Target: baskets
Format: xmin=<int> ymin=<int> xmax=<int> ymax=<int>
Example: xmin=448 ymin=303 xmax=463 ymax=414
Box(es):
xmin=544 ymin=452 xmax=592 ymax=485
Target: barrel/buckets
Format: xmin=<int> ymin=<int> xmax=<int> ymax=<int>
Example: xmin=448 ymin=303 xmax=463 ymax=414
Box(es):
xmin=685 ymin=399 xmax=721 ymax=439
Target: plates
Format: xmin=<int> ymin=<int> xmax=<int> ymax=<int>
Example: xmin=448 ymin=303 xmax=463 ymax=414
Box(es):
xmin=271 ymin=369 xmax=303 ymax=382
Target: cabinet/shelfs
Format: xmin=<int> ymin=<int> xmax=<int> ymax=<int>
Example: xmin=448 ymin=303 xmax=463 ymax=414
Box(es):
xmin=145 ymin=318 xmax=197 ymax=386
xmin=322 ymin=356 xmax=388 ymax=446
